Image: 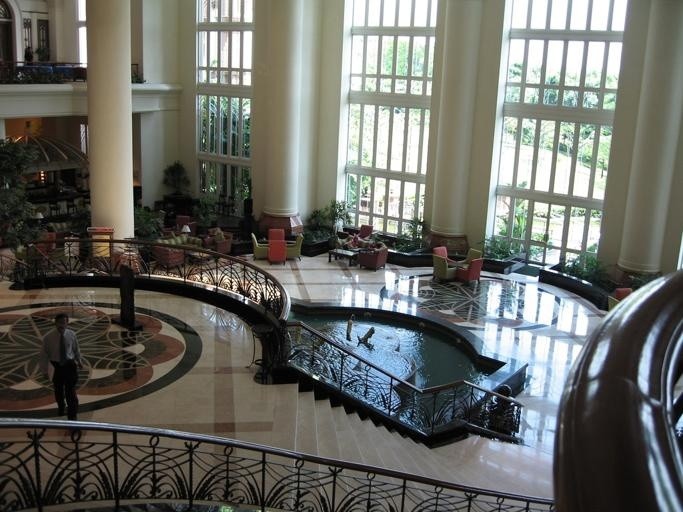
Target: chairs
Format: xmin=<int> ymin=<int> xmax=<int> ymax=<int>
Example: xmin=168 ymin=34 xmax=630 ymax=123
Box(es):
xmin=203 ymin=231 xmax=233 ymax=259
xmin=251 ymin=228 xmax=304 ymax=266
xmin=431 ymin=243 xmax=485 ymax=286
xmin=214 ymin=194 xmax=235 ymax=216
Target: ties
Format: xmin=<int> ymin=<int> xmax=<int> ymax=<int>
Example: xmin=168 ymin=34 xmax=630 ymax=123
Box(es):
xmin=60 ymin=334 xmax=66 ymax=362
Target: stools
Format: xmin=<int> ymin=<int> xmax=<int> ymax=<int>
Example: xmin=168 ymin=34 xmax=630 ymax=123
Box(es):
xmin=154 ymin=200 xmax=169 ymax=211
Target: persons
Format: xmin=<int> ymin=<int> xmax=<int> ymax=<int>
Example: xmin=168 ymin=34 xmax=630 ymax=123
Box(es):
xmin=39 ymin=314 xmax=84 ymax=422
xmin=25 ymin=46 xmax=33 ymax=66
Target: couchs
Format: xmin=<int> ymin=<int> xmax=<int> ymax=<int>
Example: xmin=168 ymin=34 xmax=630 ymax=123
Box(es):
xmin=335 ymin=236 xmax=389 ymax=272
xmin=151 ymin=235 xmax=203 ymax=269
